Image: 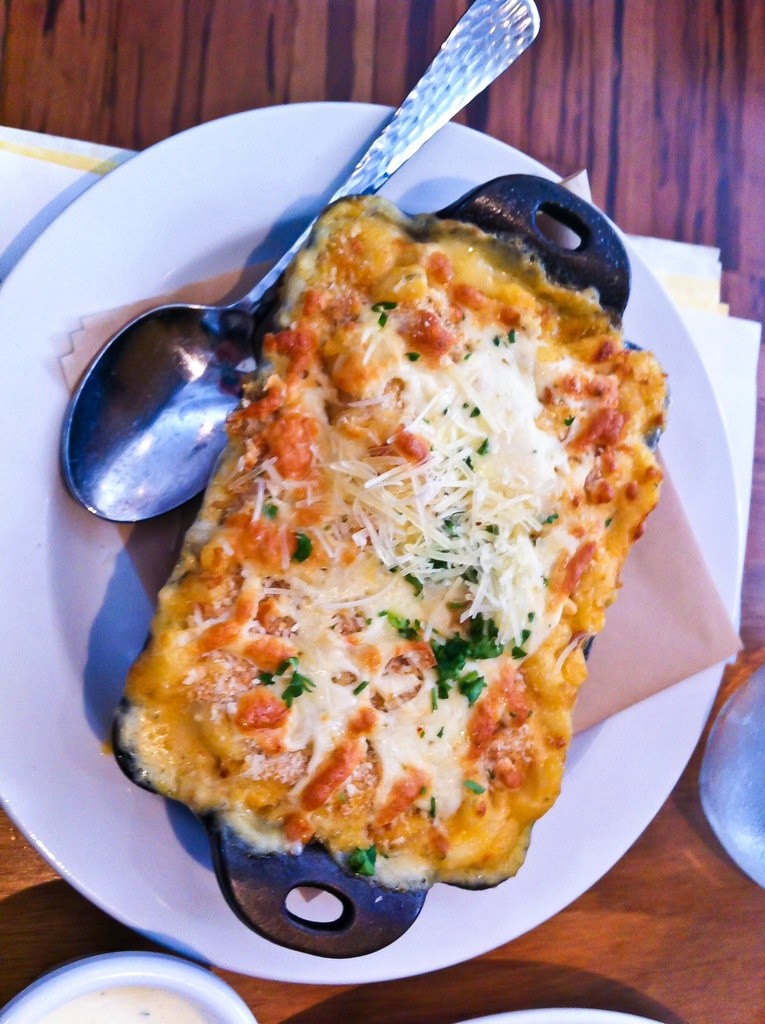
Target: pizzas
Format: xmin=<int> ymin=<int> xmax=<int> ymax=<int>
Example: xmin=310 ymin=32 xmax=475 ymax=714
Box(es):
xmin=115 ymin=192 xmax=670 ymax=885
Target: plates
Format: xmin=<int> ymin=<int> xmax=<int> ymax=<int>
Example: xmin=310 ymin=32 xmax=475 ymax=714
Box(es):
xmin=0 ymin=101 xmax=741 ymax=985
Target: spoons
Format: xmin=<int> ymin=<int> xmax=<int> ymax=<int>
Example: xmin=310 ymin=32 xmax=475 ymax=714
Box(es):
xmin=60 ymin=0 xmax=541 ymax=523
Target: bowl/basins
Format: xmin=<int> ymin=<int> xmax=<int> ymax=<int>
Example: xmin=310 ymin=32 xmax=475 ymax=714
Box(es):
xmin=0 ymin=951 xmax=259 ymax=1024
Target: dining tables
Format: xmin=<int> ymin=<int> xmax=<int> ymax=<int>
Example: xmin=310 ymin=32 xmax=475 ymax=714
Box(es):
xmin=0 ymin=0 xmax=765 ymax=1024
